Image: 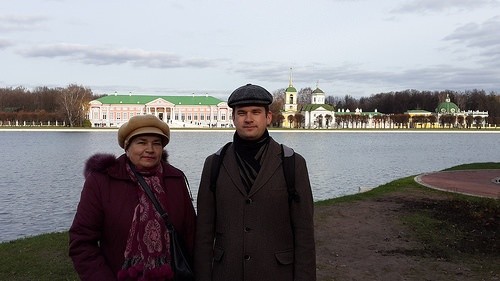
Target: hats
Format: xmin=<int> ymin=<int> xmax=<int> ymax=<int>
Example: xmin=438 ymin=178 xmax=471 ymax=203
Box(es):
xmin=118 ymin=115 xmax=171 ymax=148
xmin=227 ymin=84 xmax=273 ymax=109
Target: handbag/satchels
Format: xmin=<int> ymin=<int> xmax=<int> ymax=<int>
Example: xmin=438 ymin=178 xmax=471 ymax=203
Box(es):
xmin=171 ymin=230 xmax=193 ymax=279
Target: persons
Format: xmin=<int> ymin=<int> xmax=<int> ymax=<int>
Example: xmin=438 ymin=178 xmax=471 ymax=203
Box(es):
xmin=68 ymin=114 xmax=196 ymax=281
xmin=195 ymin=84 xmax=317 ymax=281
